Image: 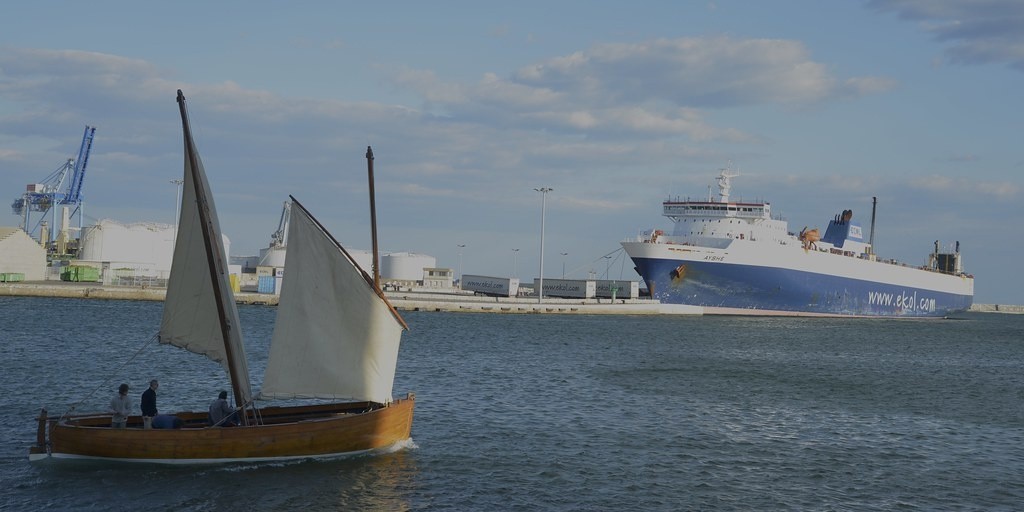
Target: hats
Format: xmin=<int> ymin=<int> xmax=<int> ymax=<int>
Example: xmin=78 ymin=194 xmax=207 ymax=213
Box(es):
xmin=119 ymin=384 xmax=128 ymax=392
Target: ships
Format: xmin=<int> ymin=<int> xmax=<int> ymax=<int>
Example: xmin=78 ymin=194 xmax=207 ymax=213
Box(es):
xmin=618 ymin=164 xmax=974 ymax=319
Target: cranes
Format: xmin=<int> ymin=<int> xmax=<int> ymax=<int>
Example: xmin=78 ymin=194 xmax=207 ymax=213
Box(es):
xmin=13 ymin=125 xmax=96 ymax=261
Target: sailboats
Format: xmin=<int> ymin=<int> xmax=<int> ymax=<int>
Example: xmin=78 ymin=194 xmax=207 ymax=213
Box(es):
xmin=29 ymin=88 xmax=416 ymax=465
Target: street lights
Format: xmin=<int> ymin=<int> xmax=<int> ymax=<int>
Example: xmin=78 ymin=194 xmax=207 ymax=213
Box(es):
xmin=534 ymin=186 xmax=554 ymax=305
xmin=170 ymin=179 xmax=185 ymax=263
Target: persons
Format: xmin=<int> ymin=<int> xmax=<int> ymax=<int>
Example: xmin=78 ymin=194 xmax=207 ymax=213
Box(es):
xmin=141 ymin=380 xmax=159 ymax=429
xmin=209 ymin=391 xmax=243 ymax=427
xmin=106 ymin=383 xmax=132 ymax=428
